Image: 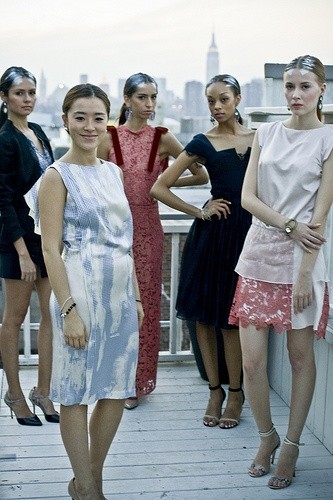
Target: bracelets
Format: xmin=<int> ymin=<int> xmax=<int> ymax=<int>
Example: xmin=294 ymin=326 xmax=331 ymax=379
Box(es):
xmin=60 ymin=297 xmax=72 ymax=312
xmin=136 ymin=300 xmax=141 ymax=302
xmin=201 ymin=209 xmax=211 ymax=220
xmin=60 ymin=303 xmax=76 ymax=317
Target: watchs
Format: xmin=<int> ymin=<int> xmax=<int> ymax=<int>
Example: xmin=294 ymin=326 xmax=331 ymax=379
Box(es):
xmin=284 ymin=219 xmax=296 ymax=235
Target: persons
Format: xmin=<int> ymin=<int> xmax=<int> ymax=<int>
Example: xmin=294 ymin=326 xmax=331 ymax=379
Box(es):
xmin=98 ymin=73 xmax=210 ymax=410
xmin=229 ymin=55 xmax=333 ymax=491
xmin=150 ymin=75 xmax=255 ymax=429
xmin=0 ymin=66 xmax=61 ymax=426
xmin=24 ymin=83 xmax=145 ymax=500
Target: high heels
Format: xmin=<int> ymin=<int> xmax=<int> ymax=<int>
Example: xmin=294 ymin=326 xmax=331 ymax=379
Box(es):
xmin=248 ymin=427 xmax=281 ymax=477
xmin=28 ymin=386 xmax=60 ymax=423
xmin=218 ymin=387 xmax=245 ymax=429
xmin=4 ymin=392 xmax=43 ymax=426
xmin=203 ymin=381 xmax=226 ymax=428
xmin=269 ymin=436 xmax=305 ymax=489
xmin=68 ymin=478 xmax=79 ymax=500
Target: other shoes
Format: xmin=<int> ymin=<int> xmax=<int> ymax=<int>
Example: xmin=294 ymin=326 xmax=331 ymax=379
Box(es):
xmin=123 ymin=398 xmax=138 ymax=409
